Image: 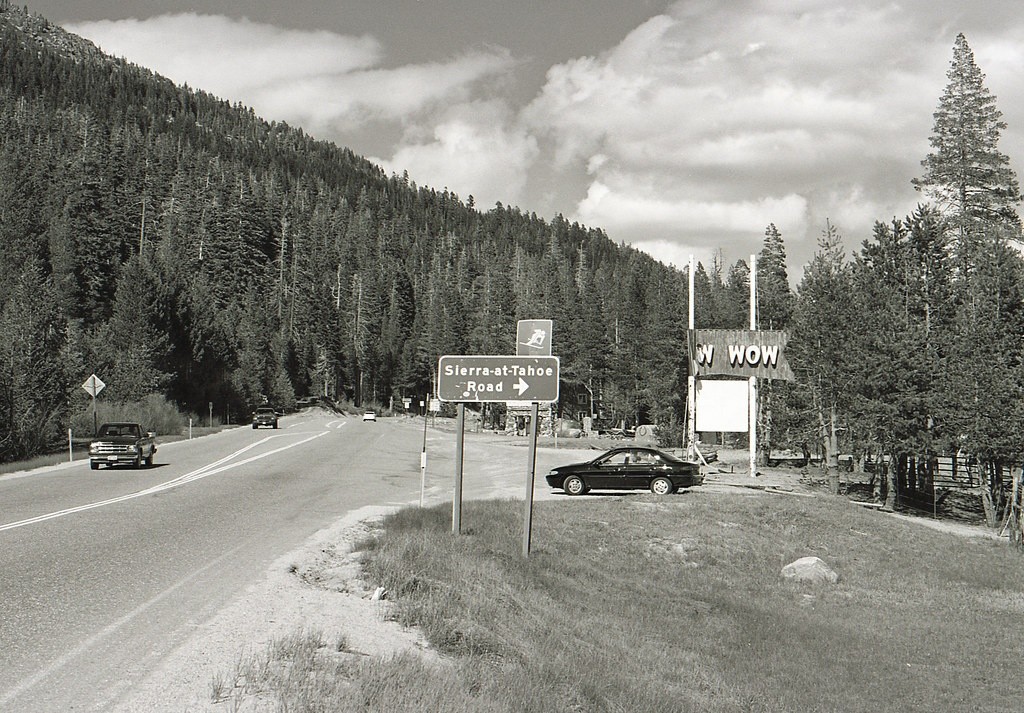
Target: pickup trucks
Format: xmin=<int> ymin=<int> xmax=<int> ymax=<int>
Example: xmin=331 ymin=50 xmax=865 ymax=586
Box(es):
xmin=85 ymin=422 xmax=158 ymax=470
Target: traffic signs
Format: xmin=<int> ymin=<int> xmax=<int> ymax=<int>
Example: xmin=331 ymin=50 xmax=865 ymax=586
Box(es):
xmin=435 ymin=352 xmax=558 ymax=405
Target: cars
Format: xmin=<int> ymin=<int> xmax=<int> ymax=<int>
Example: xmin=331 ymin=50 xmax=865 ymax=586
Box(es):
xmin=251 ymin=407 xmax=278 ymax=431
xmin=364 ymin=409 xmax=377 ymax=423
xmin=547 ymin=445 xmax=702 ymax=497
